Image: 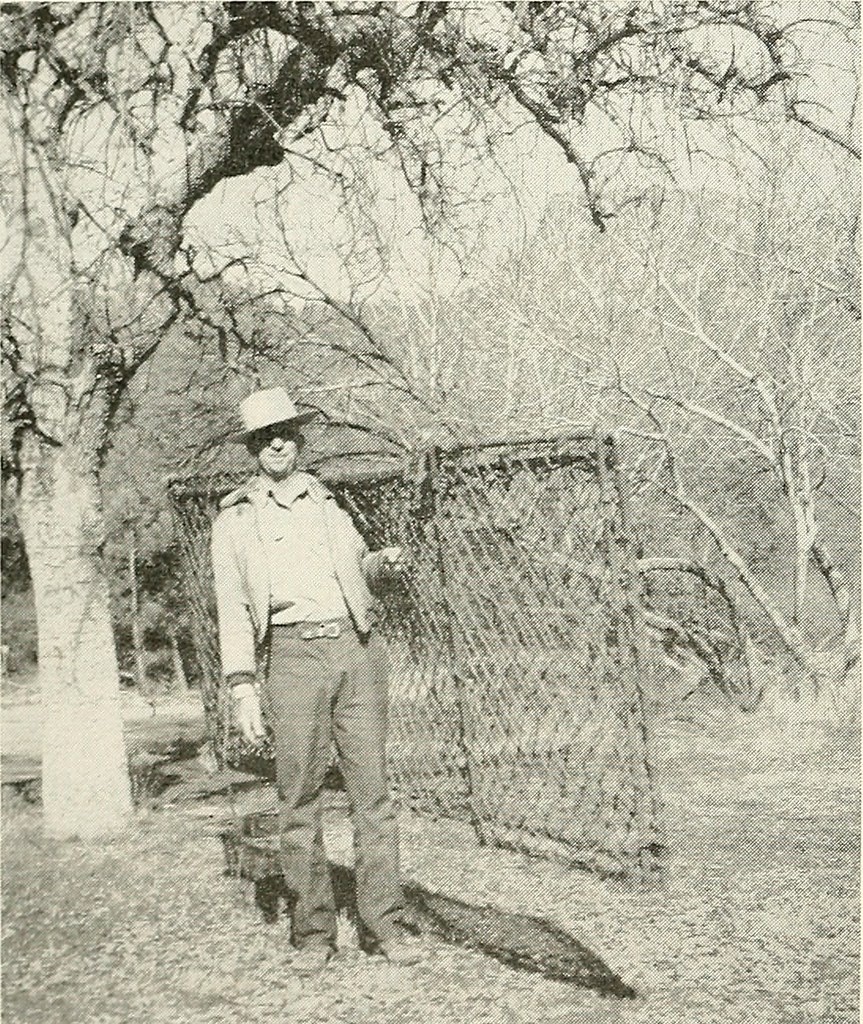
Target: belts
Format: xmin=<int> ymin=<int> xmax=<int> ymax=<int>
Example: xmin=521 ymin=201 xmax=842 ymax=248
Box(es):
xmin=266 ymin=618 xmax=354 ymax=639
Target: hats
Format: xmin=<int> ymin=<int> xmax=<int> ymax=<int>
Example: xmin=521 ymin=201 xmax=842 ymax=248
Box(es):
xmin=226 ymin=386 xmax=319 ymax=444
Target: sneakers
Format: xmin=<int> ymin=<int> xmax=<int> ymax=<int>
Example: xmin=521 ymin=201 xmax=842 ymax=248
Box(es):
xmin=372 ymin=935 xmax=423 ymax=967
xmin=291 ymin=946 xmax=336 ymax=973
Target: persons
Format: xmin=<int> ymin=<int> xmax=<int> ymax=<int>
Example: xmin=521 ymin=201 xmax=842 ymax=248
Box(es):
xmin=210 ymin=384 xmax=422 ymax=972
